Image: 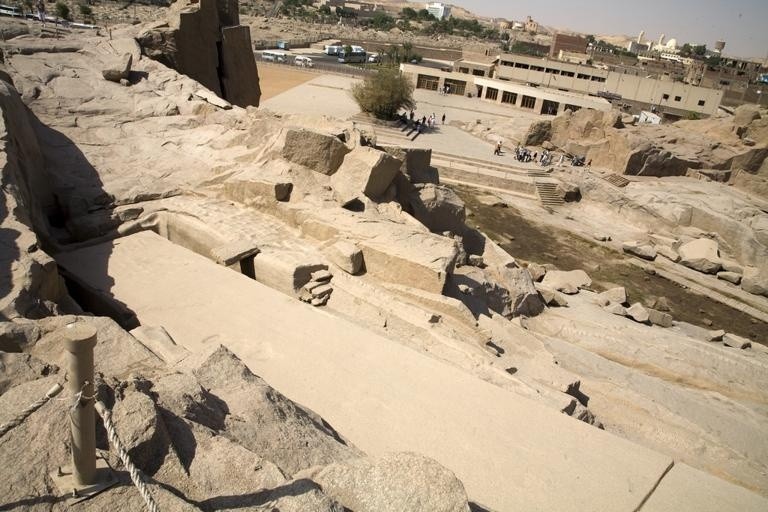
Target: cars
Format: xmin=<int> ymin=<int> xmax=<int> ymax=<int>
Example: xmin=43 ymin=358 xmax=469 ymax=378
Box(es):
xmin=755 ymin=73 xmax=767 ymax=84
xmin=368 ymin=55 xmax=383 ymax=63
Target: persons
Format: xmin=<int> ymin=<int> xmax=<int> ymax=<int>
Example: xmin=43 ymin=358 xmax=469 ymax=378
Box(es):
xmin=438 ymin=84 xmax=451 ymax=95
xmin=402 ymin=109 xmax=447 ymax=129
xmin=494 ymin=141 xmax=593 ymax=173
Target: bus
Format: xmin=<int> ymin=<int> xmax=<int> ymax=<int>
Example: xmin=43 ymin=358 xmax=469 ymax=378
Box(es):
xmin=324 ymin=45 xmax=364 ymax=56
xmin=0 ymin=4 xmax=22 ymax=18
xmin=25 ymin=13 xmax=67 ymax=28
xmin=261 ymin=50 xmax=287 ymax=64
xmin=68 ymin=22 xmax=102 ymax=32
xmin=338 ymin=51 xmax=366 ymax=64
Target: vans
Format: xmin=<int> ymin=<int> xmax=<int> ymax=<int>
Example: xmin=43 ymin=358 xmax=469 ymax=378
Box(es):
xmin=276 ymin=41 xmax=290 ymax=50
xmin=294 ymin=56 xmax=312 ymax=67
